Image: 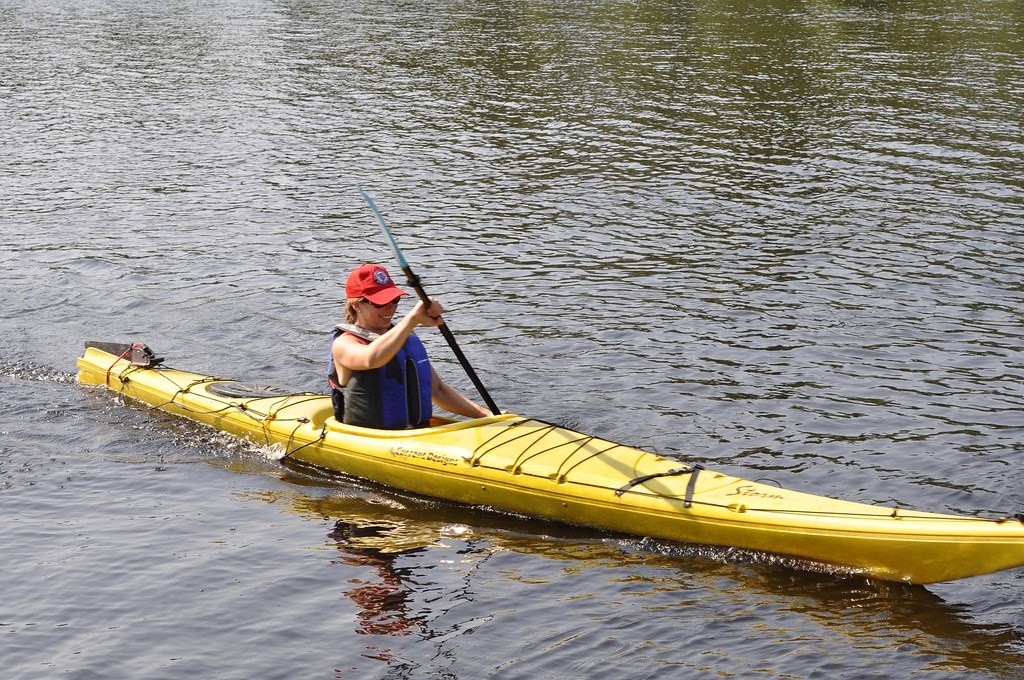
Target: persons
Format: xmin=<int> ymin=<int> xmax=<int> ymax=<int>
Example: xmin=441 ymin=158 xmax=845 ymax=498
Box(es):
xmin=328 ymin=263 xmax=508 ymax=431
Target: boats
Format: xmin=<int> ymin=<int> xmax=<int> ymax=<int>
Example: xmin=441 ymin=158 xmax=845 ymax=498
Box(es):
xmin=72 ymin=332 xmax=1024 ymax=589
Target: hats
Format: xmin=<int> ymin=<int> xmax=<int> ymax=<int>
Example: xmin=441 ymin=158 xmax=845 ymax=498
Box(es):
xmin=346 ymin=263 xmax=410 ymax=306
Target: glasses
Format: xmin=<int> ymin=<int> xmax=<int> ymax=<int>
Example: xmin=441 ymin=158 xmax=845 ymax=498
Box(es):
xmin=359 ymin=296 xmax=401 ymax=308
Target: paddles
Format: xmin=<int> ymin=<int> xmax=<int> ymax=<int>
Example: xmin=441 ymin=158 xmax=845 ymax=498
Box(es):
xmin=354 ymin=180 xmax=504 ymax=417
